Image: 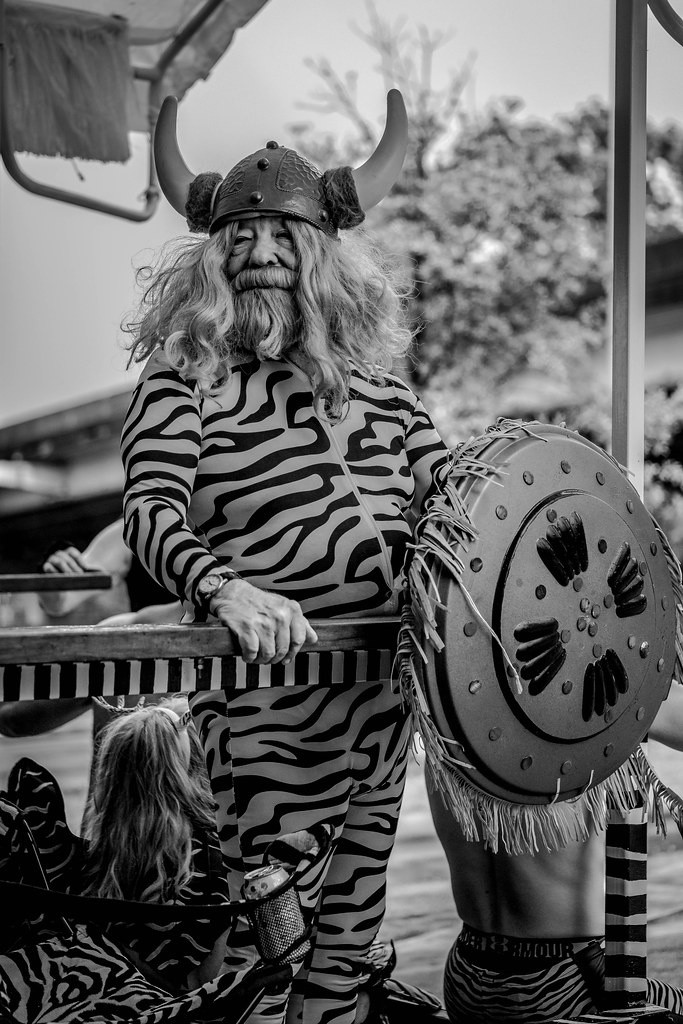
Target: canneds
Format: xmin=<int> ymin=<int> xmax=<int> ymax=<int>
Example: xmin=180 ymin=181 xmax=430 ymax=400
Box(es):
xmin=243 ymin=864 xmax=312 ymax=965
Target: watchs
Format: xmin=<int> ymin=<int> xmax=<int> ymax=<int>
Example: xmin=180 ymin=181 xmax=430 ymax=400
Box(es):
xmin=198 ymin=571 xmax=243 ymax=614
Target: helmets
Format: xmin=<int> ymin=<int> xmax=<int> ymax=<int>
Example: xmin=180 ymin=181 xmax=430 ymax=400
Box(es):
xmin=154 ymin=88 xmax=408 ymax=238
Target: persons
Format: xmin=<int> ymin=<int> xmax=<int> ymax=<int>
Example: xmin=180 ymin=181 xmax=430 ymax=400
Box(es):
xmin=0 ymin=695 xmax=233 ymax=1024
xmin=36 ymin=517 xmax=196 ymax=625
xmin=123 ymin=89 xmax=452 ymax=1024
xmin=424 ymin=678 xmax=683 ymax=1024
